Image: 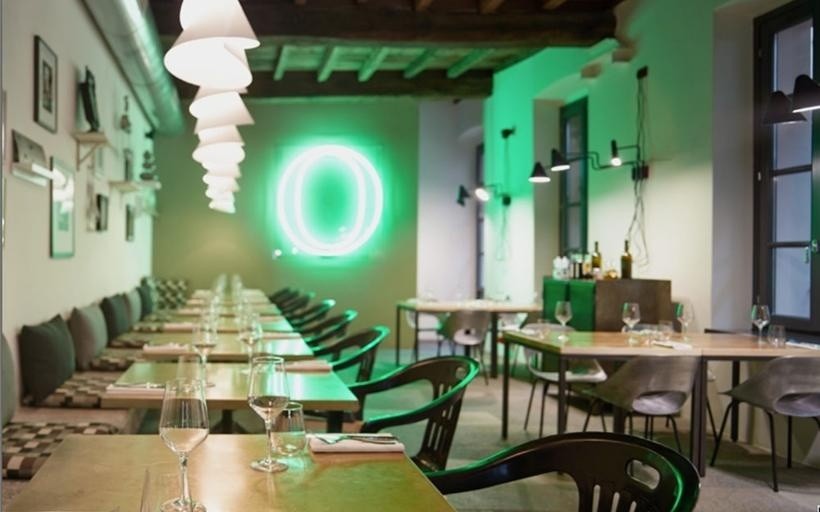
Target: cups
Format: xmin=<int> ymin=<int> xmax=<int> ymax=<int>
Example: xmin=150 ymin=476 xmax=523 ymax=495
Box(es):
xmin=271 ymin=401 xmax=307 ymax=456
xmin=768 ymin=325 xmax=786 ymax=347
xmin=537 ymin=319 xmax=551 ymax=339
xmin=659 ymin=320 xmax=673 ymax=341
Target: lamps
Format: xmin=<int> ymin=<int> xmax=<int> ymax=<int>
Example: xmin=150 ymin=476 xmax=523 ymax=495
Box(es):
xmin=162 ymin=0 xmax=261 ymax=214
xmin=457 ymin=139 xmax=648 ymax=206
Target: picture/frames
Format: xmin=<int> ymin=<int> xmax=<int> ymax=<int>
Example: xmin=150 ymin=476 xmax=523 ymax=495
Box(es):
xmin=96 ymin=193 xmax=109 ymax=232
xmin=49 ymin=156 xmax=76 ymax=258
xmin=34 ymin=35 xmax=58 ymax=133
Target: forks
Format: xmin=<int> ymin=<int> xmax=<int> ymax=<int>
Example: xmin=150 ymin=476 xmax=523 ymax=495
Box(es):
xmin=315 ymin=434 xmax=398 ymax=445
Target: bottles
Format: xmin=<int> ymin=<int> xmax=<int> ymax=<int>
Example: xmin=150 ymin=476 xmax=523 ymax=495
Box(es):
xmin=621 ymin=240 xmax=632 ymax=279
xmin=553 ymin=253 xmax=590 ymax=280
xmin=591 ymin=241 xmax=602 ymax=272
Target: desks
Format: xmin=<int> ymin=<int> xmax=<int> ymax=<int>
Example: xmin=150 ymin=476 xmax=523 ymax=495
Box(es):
xmin=542 ymin=274 xmax=671 ymax=416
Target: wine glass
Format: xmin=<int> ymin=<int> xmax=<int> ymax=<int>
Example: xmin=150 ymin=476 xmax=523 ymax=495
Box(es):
xmin=677 ymin=303 xmax=695 ymax=343
xmin=191 ymin=320 xmax=216 ymax=388
xmin=199 ymin=274 xmax=263 ymax=376
xmin=751 ymin=305 xmax=770 ymax=345
xmin=555 ymin=302 xmax=572 ymax=341
xmin=159 ymin=377 xmax=209 ymax=512
xmin=247 ymin=356 xmax=289 ymax=472
xmin=621 ymin=302 xmax=641 ymax=345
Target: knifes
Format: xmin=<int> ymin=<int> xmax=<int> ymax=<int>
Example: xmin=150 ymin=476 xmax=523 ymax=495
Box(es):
xmin=324 ymin=437 xmax=397 ymax=446
